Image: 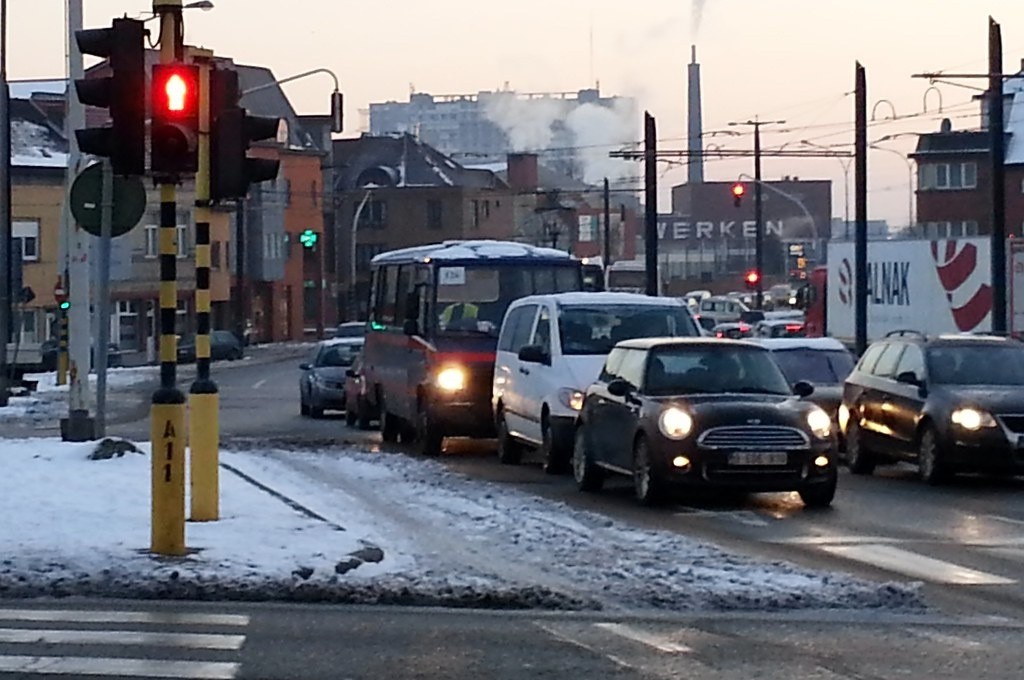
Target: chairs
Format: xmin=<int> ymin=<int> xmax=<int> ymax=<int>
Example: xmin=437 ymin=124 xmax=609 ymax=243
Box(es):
xmin=569 ymin=323 xmax=592 ymax=339
xmin=609 ymin=325 xmax=626 ymax=337
xmin=931 ymin=354 xmax=957 ymax=375
xmin=648 ymin=359 xmax=666 ymax=389
xmin=322 ymin=350 xmax=342 ymax=364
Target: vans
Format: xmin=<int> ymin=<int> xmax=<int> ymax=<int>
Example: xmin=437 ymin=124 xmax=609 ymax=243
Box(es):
xmin=494 ymin=292 xmax=711 ymax=477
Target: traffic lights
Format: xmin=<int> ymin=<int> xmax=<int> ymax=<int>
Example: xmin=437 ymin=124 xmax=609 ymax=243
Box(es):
xmin=150 ymin=63 xmax=202 ymax=175
xmin=733 ymin=181 xmax=744 ymax=207
xmin=60 ymin=302 xmax=70 ymax=313
xmin=747 ymin=271 xmax=758 ymax=287
xmin=74 ymin=14 xmax=146 ymax=175
xmin=210 ymin=67 xmax=280 ymax=202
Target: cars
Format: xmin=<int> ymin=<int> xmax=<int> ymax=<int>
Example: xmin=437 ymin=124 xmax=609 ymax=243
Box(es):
xmin=297 ymin=337 xmax=365 ymax=418
xmin=341 ymin=351 xmax=363 ymax=426
xmin=685 ymin=282 xmax=808 ymax=341
xmin=740 ymin=337 xmax=861 ymax=437
xmin=330 ymin=320 xmax=367 ymax=342
xmin=177 ymin=329 xmax=244 ymax=364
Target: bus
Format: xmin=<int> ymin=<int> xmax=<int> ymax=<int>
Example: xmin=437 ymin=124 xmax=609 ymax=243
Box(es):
xmin=361 ymin=240 xmax=608 ymax=457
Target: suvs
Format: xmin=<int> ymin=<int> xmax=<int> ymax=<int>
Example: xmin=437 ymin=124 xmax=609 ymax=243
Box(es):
xmin=572 ymin=338 xmax=836 ymax=508
xmin=835 ymin=329 xmax=1024 ymax=487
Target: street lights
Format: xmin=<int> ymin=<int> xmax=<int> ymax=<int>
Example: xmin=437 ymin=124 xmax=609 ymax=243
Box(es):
xmin=729 ymin=117 xmax=788 ymax=309
xmin=60 ymin=0 xmax=217 ymax=441
